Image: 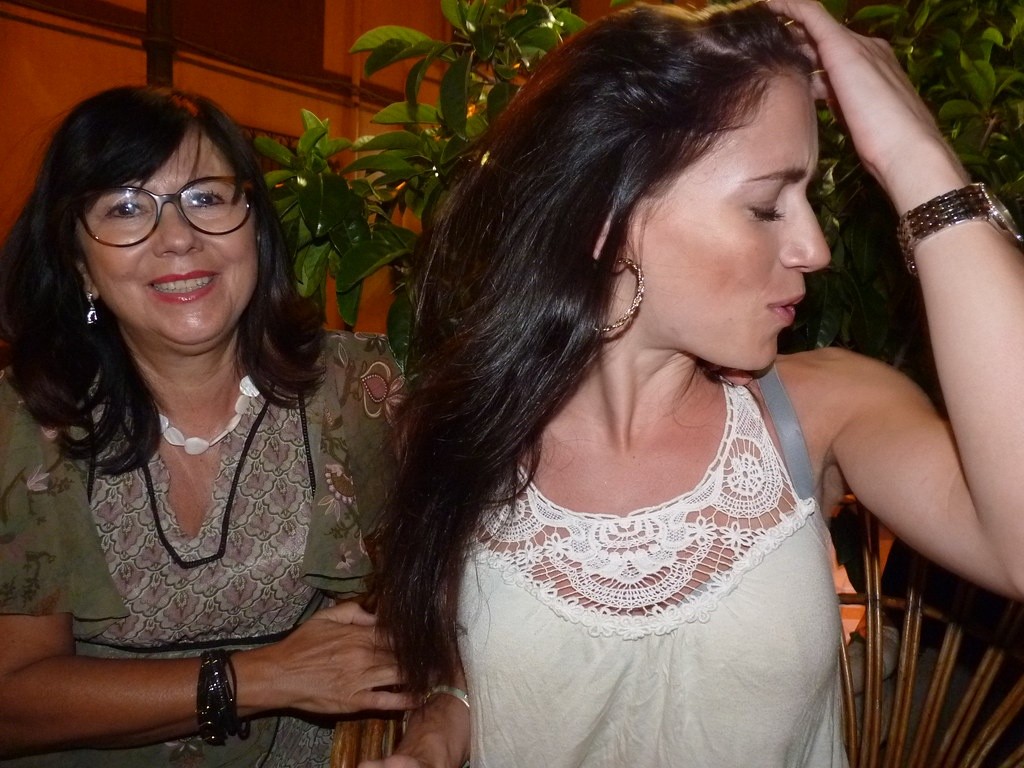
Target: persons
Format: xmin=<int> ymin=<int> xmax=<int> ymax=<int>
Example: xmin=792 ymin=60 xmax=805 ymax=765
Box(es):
xmin=0 ymin=84 xmax=470 ymax=768
xmin=386 ymin=0 xmax=1023 ymax=766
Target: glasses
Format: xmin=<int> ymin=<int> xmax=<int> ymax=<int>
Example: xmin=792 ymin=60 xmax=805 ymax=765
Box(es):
xmin=75 ymin=175 xmax=252 ymax=247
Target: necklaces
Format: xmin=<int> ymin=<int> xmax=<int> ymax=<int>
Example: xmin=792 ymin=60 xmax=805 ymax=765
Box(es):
xmin=150 ymin=376 xmax=260 ymax=454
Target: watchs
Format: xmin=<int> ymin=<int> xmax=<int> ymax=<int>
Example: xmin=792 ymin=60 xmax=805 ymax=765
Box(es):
xmin=897 ymin=182 xmax=1023 ymax=273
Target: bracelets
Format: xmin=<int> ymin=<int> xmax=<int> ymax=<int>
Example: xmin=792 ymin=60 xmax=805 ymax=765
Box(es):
xmin=422 ymin=685 xmax=472 ymax=711
xmin=195 ymin=648 xmax=238 ymax=747
